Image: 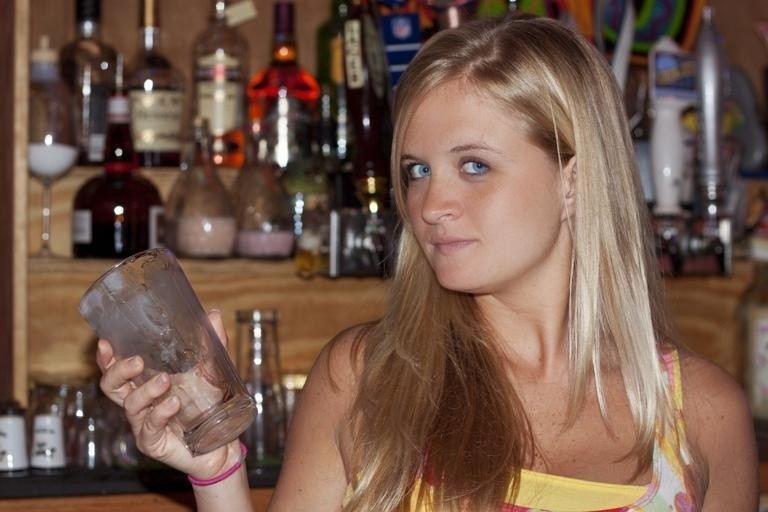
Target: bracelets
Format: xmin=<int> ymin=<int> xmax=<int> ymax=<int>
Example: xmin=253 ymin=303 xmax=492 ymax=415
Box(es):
xmin=186 ymin=441 xmax=248 ymax=489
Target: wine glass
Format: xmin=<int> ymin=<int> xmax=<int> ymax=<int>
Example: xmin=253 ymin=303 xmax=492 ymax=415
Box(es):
xmin=27 ymin=91 xmax=82 ymax=258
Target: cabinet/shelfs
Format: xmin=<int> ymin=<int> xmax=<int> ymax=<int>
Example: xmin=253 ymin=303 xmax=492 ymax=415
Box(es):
xmin=1 ymin=2 xmax=767 ymax=411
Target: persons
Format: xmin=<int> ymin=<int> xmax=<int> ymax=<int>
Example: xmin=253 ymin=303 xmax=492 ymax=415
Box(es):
xmin=92 ymin=8 xmax=760 ymax=512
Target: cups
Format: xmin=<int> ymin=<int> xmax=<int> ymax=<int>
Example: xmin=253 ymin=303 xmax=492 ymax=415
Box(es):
xmin=77 ymin=245 xmax=258 ymax=457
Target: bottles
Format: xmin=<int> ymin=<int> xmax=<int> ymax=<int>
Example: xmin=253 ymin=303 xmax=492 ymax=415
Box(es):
xmin=249 ymin=1 xmax=318 ymax=163
xmin=63 ymin=2 xmax=112 ymax=162
xmin=237 ymin=119 xmax=296 ymax=257
xmin=342 ymin=165 xmax=398 ymax=279
xmin=231 ymin=305 xmax=287 ymax=488
xmin=109 ymin=51 xmax=132 ymax=163
xmin=164 ymin=112 xmax=237 ymax=257
xmin=301 ymin=190 xmax=330 ymax=275
xmin=0 ymin=383 xmax=111 ymax=476
xmin=123 ymin=2 xmax=185 ymax=168
xmin=321 ymin=1 xmax=384 ymax=157
xmin=76 ymin=164 xmax=164 ymax=255
xmin=192 ymin=0 xmax=246 ymax=167
